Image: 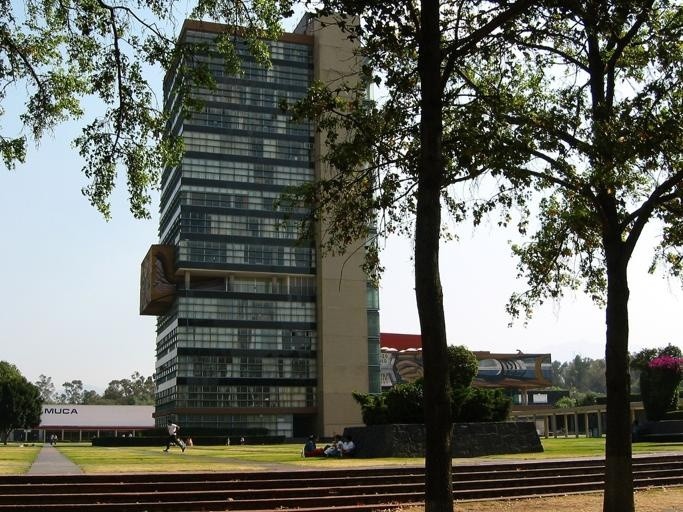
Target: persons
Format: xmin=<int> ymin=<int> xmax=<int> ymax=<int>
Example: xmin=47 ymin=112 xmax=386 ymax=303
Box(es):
xmin=240 ymin=434 xmax=245 ymax=446
xmin=50 ymin=433 xmax=54 ymax=445
xmin=225 ymin=436 xmax=230 ymax=446
xmin=186 ymin=435 xmax=194 ymax=446
xmin=305 ymin=432 xmax=356 ymax=458
xmin=128 ymin=431 xmax=132 ymax=438
xmin=121 ymin=432 xmax=126 ymax=438
xmin=53 ymin=433 xmax=58 ymax=444
xmin=164 ymin=419 xmax=186 ymax=454
